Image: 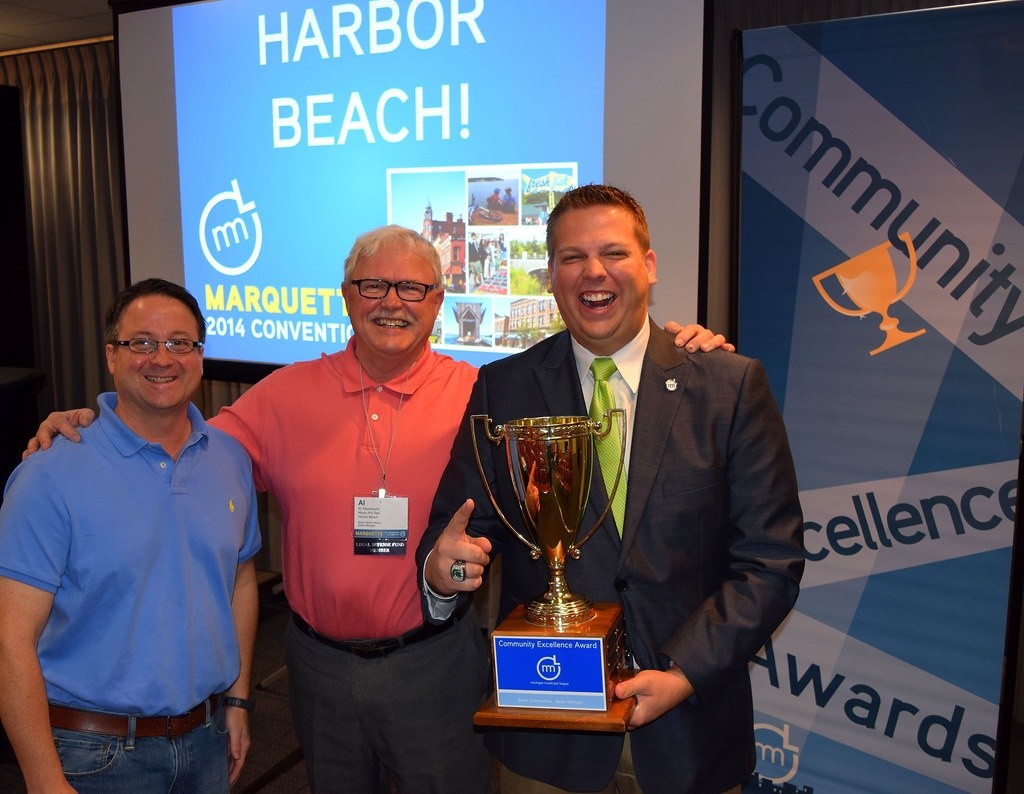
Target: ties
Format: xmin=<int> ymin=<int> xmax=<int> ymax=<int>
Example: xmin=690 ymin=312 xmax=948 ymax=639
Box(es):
xmin=589 ymin=358 xmax=628 ymax=542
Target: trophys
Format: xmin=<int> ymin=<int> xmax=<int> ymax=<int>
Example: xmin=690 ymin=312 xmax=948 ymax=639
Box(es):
xmin=468 ymin=408 xmax=641 ymax=734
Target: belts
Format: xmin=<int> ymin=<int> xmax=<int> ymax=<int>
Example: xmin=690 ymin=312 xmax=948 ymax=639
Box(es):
xmin=48 ymin=695 xmax=220 ymax=740
xmin=290 ymin=595 xmax=473 ymax=658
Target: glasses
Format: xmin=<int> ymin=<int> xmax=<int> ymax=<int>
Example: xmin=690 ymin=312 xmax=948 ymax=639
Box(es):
xmin=110 ymin=338 xmax=202 ymax=353
xmin=350 ymin=279 xmax=438 ymax=302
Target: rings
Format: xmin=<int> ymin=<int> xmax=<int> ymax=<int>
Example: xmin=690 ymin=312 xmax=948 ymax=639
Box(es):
xmin=450 ymin=561 xmax=467 ymax=582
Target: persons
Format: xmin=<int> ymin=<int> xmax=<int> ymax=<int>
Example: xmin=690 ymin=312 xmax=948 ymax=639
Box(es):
xmin=487 ymin=187 xmax=518 ymax=215
xmin=468 ymin=231 xmax=507 ymax=288
xmin=18 ymin=224 xmax=737 ymax=794
xmin=409 ymin=181 xmax=803 ymax=794
xmin=0 ymin=278 xmax=266 ymax=794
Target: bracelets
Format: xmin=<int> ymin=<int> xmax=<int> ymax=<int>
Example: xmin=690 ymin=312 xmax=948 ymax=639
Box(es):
xmin=222 ymin=695 xmax=254 ymax=713
